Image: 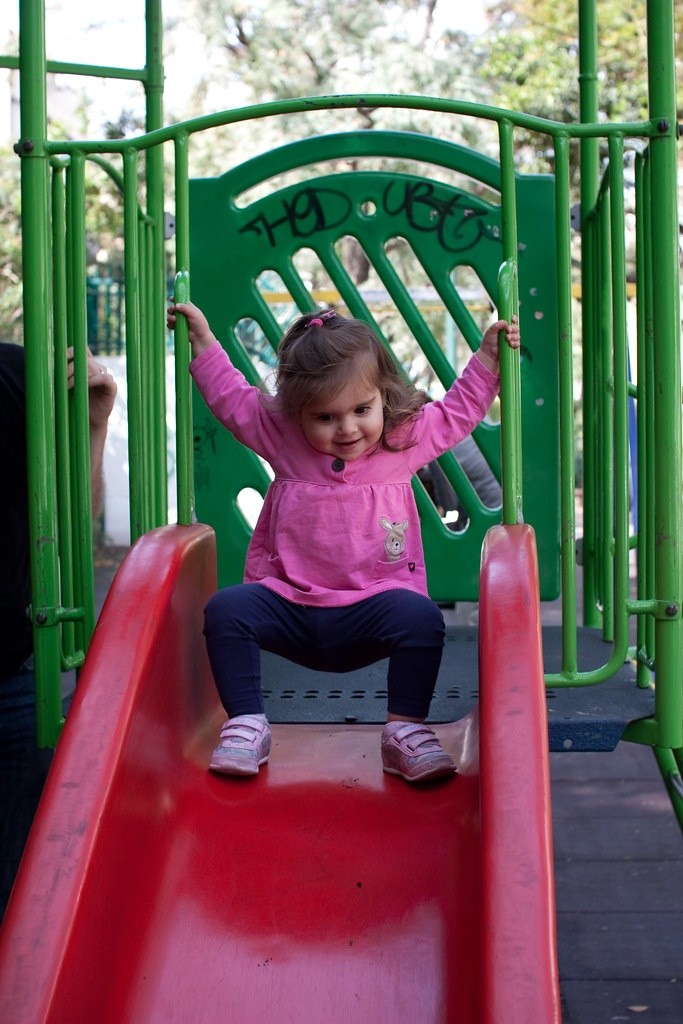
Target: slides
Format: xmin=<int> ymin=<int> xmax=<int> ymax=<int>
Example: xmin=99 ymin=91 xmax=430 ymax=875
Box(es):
xmin=0 ymin=518 xmax=569 ymax=1024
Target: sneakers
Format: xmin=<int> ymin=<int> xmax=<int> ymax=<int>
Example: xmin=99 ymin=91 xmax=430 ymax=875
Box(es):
xmin=380 ymin=724 xmax=457 ymax=781
xmin=210 ymin=714 xmax=271 ymax=776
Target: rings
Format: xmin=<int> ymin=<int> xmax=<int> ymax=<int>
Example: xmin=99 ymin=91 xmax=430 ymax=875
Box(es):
xmin=99 ymin=368 xmax=105 ymax=374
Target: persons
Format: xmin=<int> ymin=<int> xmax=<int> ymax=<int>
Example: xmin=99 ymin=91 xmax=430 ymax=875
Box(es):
xmin=416 ymin=432 xmax=505 ymax=610
xmin=166 ymin=295 xmax=521 ymax=783
xmin=0 ymin=342 xmax=118 ymax=926
